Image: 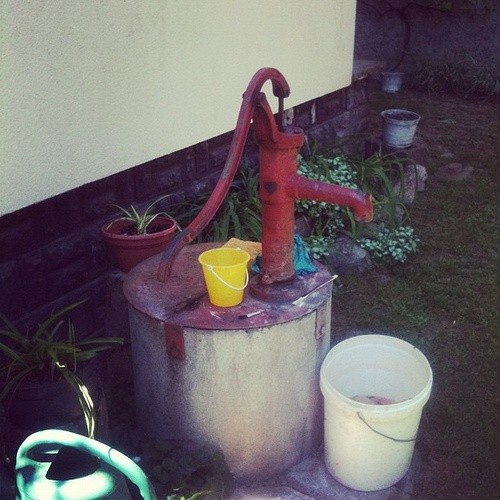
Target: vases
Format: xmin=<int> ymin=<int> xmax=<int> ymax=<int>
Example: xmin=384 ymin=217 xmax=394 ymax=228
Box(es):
xmin=381 ymin=108 xmax=421 ymax=148
xmin=295 ymin=215 xmax=312 ymax=236
xmin=383 ymin=71 xmax=403 ymax=92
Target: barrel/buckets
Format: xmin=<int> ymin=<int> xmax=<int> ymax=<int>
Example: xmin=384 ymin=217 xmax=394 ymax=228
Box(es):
xmin=198 ymin=246 xmax=250 ymax=307
xmin=318 ymin=334 xmax=433 ymax=492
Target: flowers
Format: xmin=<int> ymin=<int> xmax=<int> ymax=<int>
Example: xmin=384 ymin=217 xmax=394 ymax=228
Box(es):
xmin=294 ymin=155 xmax=423 ymax=265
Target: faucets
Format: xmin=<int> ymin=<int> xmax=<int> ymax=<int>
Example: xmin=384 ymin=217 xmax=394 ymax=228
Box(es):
xmin=284 ymin=173 xmax=374 ymax=224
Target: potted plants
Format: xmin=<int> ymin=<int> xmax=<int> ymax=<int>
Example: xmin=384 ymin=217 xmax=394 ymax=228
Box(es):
xmin=1 ymin=295 xmax=124 ymax=438
xmin=99 ymin=192 xmax=178 ymax=267
